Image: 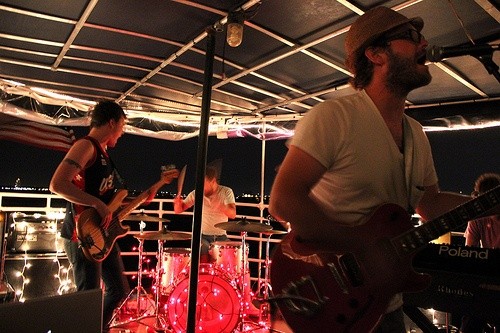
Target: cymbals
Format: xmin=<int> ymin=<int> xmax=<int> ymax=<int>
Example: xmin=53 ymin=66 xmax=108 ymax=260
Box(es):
xmin=122 ymin=214 xmax=171 ymax=223
xmin=260 ymin=230 xmax=287 ymax=236
xmin=133 ymin=228 xmax=192 ymax=240
xmin=213 ymin=221 xmax=273 ymax=232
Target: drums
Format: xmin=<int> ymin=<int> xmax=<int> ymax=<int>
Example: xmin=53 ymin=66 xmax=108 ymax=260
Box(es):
xmin=210 ymin=240 xmax=251 ymax=276
xmin=165 ymin=264 xmax=243 ymax=333
xmin=151 ymin=248 xmax=192 ymax=294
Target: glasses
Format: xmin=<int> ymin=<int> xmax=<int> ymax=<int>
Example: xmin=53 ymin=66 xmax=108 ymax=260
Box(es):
xmin=378 ymin=29 xmax=427 ymax=46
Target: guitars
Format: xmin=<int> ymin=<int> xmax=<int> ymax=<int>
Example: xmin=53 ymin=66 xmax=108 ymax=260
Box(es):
xmin=270 ymin=184 xmax=500 ymax=333
xmin=75 ymin=163 xmax=179 ymax=262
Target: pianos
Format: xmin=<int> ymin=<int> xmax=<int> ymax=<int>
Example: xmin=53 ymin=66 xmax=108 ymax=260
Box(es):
xmin=400 ymin=243 xmax=500 ymax=333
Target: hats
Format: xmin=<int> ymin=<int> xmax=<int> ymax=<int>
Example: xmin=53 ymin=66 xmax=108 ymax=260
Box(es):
xmin=344 ymin=6 xmax=424 ymax=59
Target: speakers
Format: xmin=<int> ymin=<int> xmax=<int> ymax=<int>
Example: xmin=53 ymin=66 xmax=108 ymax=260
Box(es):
xmin=409 ymin=268 xmax=500 ymax=333
xmin=1 ymin=288 xmax=102 ymax=332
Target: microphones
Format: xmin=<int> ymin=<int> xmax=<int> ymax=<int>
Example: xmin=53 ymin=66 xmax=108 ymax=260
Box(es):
xmin=427 ymin=43 xmax=500 ymax=62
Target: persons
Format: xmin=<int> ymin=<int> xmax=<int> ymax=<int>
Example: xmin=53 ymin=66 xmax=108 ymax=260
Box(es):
xmin=49 ymin=99 xmax=157 ymax=333
xmin=173 ymin=167 xmax=237 ymax=263
xmin=268 ymin=6 xmax=500 ymax=333
xmin=463 ymin=172 xmax=500 ymax=249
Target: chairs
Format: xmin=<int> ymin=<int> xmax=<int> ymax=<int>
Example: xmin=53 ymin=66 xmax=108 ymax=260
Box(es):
xmin=0 ymin=288 xmax=104 ymax=333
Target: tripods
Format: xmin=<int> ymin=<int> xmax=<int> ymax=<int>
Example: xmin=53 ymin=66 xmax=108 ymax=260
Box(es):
xmin=236 ymin=230 xmax=284 ymax=333
xmin=109 ymin=222 xmax=166 ymax=328
